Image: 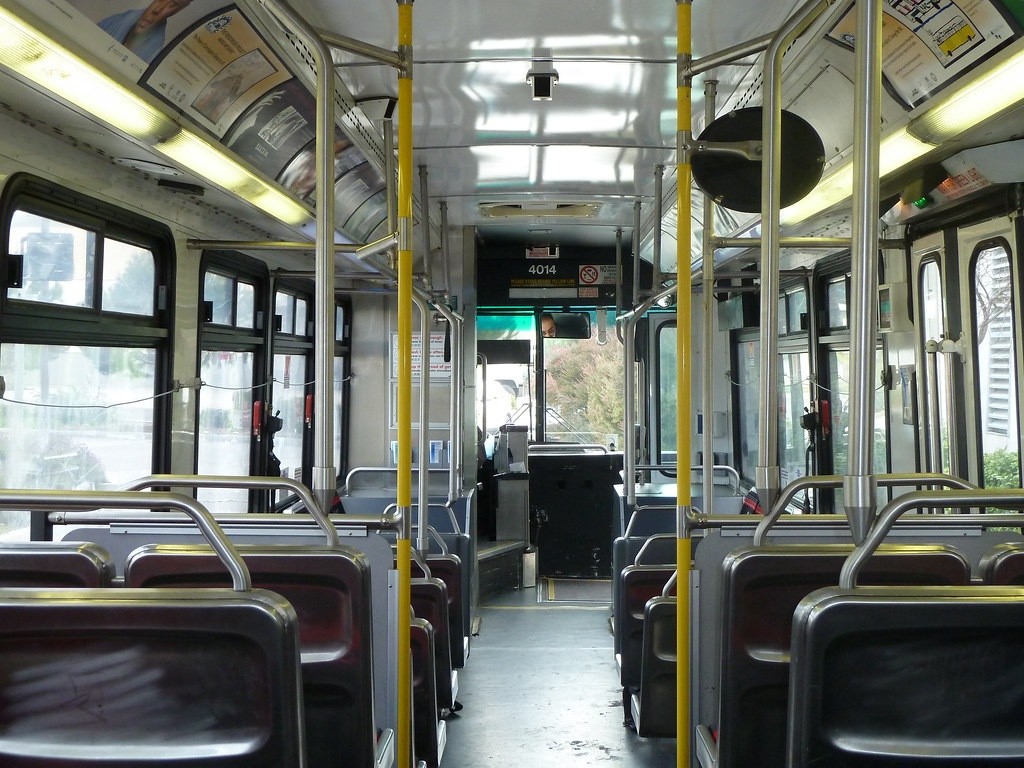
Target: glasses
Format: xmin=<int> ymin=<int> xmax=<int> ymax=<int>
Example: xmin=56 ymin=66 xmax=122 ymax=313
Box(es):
xmin=542 ymin=327 xmax=554 ymax=335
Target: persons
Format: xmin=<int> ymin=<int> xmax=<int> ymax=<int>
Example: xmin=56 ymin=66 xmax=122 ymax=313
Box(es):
xmin=541 ymin=311 xmax=589 ymax=338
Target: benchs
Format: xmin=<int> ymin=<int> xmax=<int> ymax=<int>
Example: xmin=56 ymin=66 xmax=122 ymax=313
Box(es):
xmin=606 ymin=474 xmax=1024 ymax=768
xmin=0 ymin=464 xmax=472 ymax=768
